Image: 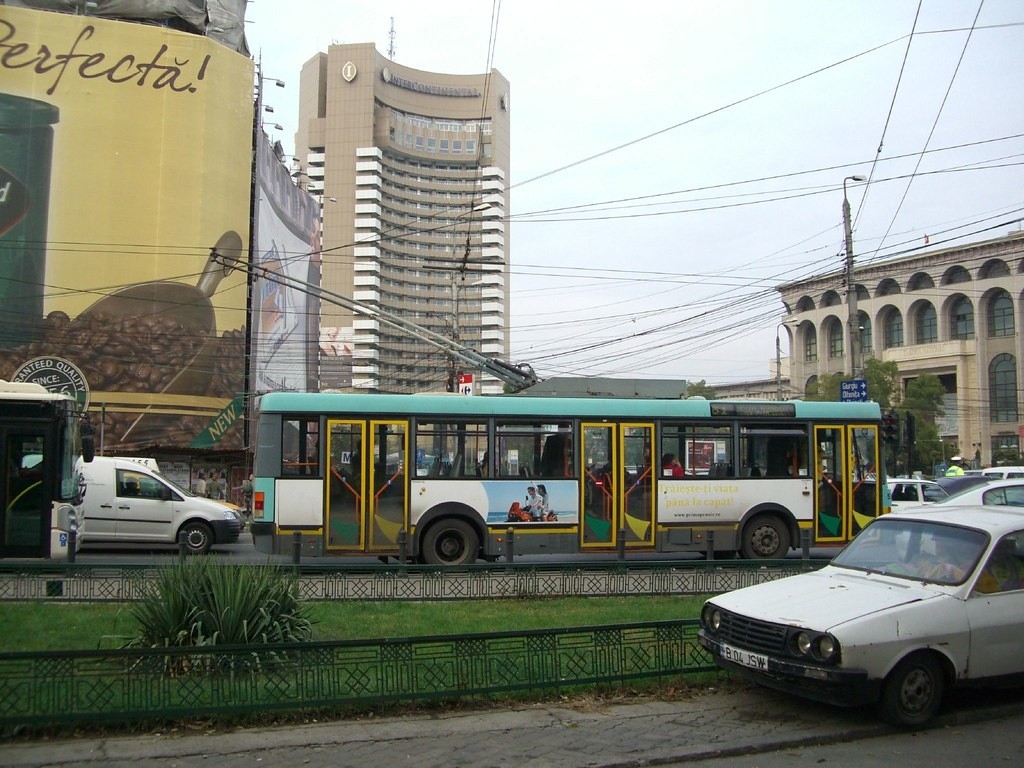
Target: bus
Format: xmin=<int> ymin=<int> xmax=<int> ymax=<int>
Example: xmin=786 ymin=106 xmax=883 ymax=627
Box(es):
xmin=0 ymin=380 xmax=97 ymax=561
xmin=211 ymin=252 xmax=892 ymax=573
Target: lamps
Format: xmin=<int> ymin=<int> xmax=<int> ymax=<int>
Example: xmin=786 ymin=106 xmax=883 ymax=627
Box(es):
xmin=321 ymin=197 xmax=336 ymax=202
xmin=262 ymin=104 xmax=274 ymax=113
xmin=263 ymin=77 xmax=286 ymax=88
xmin=263 ymin=123 xmax=283 ymax=130
xmin=284 ymin=154 xmax=301 ymax=162
xmin=297 ymin=181 xmax=315 ymax=188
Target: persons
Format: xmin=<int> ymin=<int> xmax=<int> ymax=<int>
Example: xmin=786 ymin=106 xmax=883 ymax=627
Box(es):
xmin=196 ymin=471 xmax=227 ymax=501
xmin=946 ymin=456 xmax=964 ymax=476
xmin=123 ymin=476 xmax=138 ymax=497
xmin=310 ymin=424 xmax=684 ymax=480
xmin=815 ymin=441 xmax=825 ymax=510
xmin=892 ymin=484 xmax=925 ymax=501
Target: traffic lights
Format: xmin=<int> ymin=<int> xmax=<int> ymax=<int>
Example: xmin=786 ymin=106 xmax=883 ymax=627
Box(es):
xmin=882 ymin=410 xmax=901 ymax=447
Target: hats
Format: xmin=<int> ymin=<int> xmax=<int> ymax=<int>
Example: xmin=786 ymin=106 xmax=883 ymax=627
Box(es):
xmin=951 ymin=457 xmax=962 ymax=461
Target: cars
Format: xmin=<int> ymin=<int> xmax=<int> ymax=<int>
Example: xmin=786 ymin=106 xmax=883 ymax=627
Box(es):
xmin=853 ymin=466 xmax=1024 ymax=562
xmin=697 ymin=505 xmax=1024 ymax=726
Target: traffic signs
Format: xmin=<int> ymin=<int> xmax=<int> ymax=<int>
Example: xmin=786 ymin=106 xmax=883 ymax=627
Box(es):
xmin=841 ymin=381 xmax=868 ymax=402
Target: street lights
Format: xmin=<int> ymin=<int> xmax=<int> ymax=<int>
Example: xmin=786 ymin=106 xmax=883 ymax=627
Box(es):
xmin=449 ymin=204 xmax=491 ymax=392
xmin=842 ymin=174 xmax=867 ymax=380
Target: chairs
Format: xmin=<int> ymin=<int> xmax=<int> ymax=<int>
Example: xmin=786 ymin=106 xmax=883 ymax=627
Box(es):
xmin=430 ymin=453 xmax=483 ymax=477
xmin=307 ymin=456 xmax=318 ymax=474
xmin=517 ymin=465 xmax=532 ymax=476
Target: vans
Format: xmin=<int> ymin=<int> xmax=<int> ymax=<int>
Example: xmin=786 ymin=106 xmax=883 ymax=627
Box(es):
xmin=22 ymin=455 xmax=246 ymax=555
